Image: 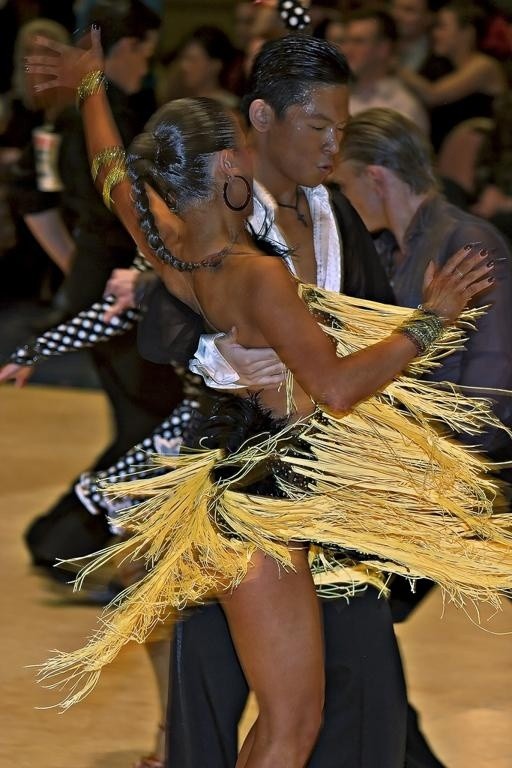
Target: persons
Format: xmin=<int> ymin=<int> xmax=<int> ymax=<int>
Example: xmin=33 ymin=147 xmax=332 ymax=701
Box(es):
xmin=20 ymin=18 xmax=511 ymax=765
xmin=137 ymin=33 xmax=411 ymax=766
xmin=2 ymin=255 xmax=202 ymax=763
xmin=0 ymin=0 xmax=512 ymax=605
xmin=330 ymin=106 xmax=512 ymax=766
